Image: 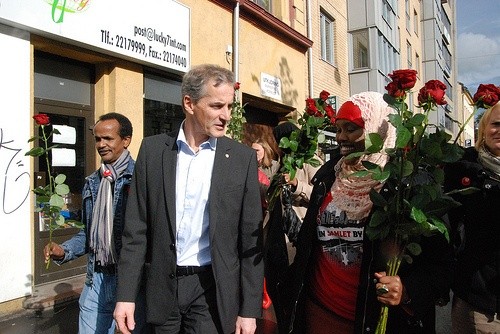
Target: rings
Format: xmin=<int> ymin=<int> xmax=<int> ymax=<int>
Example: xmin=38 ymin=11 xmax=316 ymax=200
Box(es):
xmin=378 ymin=284 xmax=389 ymax=294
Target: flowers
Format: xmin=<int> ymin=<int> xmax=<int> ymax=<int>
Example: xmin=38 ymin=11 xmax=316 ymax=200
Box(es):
xmin=344 ymin=69 xmax=500 ymax=334
xmin=264 ymin=91 xmax=337 ymax=213
xmin=226 ymin=82 xmax=248 ymax=142
xmin=24 ymin=112 xmax=85 ymax=268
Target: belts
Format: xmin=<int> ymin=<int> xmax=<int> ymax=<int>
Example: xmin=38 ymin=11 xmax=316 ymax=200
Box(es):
xmin=176 ymin=264 xmax=210 ymax=279
xmin=94 ymin=261 xmax=117 ymax=274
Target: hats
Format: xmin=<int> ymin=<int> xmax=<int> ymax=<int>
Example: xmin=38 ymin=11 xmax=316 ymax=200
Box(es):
xmin=333 ymin=96 xmax=381 ymax=130
xmin=274 ymin=123 xmax=304 ymax=155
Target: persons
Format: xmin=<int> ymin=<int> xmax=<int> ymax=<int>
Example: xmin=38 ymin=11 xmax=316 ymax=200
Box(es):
xmin=284 ymin=92 xmax=452 ymax=334
xmin=43 ymin=112 xmax=135 ymax=334
xmin=112 ymin=64 xmax=266 ymax=334
xmin=241 ymin=121 xmax=324 ymax=334
xmin=443 ymin=100 xmax=500 ymax=334
